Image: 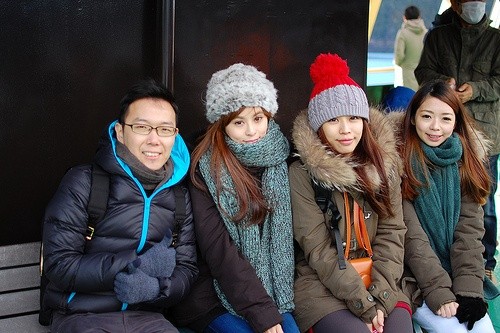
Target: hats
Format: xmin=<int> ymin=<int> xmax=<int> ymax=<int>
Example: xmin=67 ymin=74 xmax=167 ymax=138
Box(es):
xmin=205 ymin=63 xmax=279 ymax=123
xmin=307 ymin=52 xmax=369 ymax=132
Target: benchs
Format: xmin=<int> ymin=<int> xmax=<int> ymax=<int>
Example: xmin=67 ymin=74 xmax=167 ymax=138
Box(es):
xmin=0 ymin=242 xmax=52 ymax=333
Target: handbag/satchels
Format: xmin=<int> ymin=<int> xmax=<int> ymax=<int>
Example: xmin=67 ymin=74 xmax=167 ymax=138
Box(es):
xmin=347 ymin=257 xmax=373 ymax=289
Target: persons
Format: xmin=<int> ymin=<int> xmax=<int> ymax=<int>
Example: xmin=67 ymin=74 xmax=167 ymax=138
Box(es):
xmin=285 ymin=58 xmax=414 ymax=332
xmin=37 ymin=80 xmax=283 ymax=333
xmin=392 ymin=7 xmax=431 ymax=92
xmin=392 ymin=79 xmax=496 ymax=333
xmin=189 ymin=64 xmax=302 ymax=332
xmin=417 ymin=0 xmax=500 ymax=284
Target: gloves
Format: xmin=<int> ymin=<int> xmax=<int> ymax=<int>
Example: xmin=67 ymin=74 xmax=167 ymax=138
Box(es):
xmin=454 ymin=293 xmax=488 ymax=331
xmin=114 ymin=263 xmax=160 ymax=304
xmin=137 ymin=227 xmax=176 ymax=278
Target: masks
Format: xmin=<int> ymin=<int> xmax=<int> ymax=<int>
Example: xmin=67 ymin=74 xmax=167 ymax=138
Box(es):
xmin=452 ymin=1 xmax=485 ymax=25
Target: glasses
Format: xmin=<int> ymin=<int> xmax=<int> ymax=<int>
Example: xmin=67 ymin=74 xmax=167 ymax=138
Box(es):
xmin=123 ymin=123 xmax=176 ymax=137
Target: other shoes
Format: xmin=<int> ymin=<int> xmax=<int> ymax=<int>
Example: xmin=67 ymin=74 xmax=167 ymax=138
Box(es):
xmin=484 ymin=269 xmax=496 ymax=285
